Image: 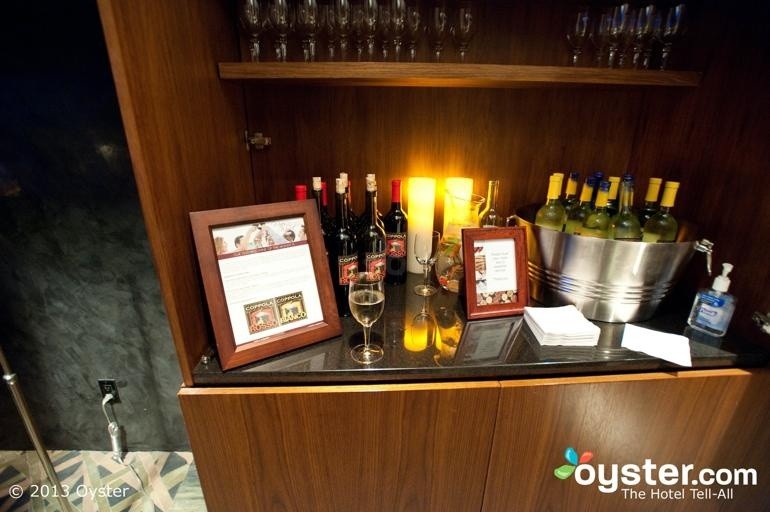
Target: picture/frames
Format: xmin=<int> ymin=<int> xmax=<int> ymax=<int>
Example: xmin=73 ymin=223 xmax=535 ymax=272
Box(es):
xmin=189 ymin=199 xmax=343 ymax=373
xmin=462 ymin=226 xmax=530 ymax=320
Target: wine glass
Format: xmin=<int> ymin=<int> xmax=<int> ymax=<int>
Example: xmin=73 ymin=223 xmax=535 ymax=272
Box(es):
xmin=347 ymin=272 xmax=387 ymax=364
xmin=409 ymin=298 xmax=436 ymax=348
xmin=231 ymin=0 xmax=476 ymax=64
xmin=412 ymin=232 xmax=440 ymax=296
xmin=565 ymin=0 xmax=683 ymax=70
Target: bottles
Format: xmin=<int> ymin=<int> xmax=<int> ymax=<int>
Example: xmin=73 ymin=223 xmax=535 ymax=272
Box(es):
xmin=435 ymin=190 xmax=485 ymax=294
xmin=294 ymin=172 xmax=406 ymax=288
xmin=533 ymin=167 xmax=683 ymax=245
xmin=479 ymin=181 xmax=505 ymax=228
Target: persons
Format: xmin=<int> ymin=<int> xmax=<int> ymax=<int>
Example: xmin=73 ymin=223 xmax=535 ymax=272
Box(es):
xmin=215 ymin=223 xmax=307 ymax=254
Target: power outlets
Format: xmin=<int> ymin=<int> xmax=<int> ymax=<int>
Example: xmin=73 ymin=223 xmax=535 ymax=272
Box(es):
xmin=98 ymin=381 xmax=121 ymax=405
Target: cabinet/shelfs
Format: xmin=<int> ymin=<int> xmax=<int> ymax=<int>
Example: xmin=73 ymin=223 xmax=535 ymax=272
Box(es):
xmin=96 ymin=0 xmax=770 ymax=512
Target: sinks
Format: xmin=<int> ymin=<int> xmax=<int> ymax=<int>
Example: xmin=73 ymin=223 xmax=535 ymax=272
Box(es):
xmin=507 ymin=204 xmax=696 ymax=323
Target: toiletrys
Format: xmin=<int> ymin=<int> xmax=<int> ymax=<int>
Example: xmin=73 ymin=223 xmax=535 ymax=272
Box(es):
xmin=687 ymin=263 xmax=736 ymax=337
